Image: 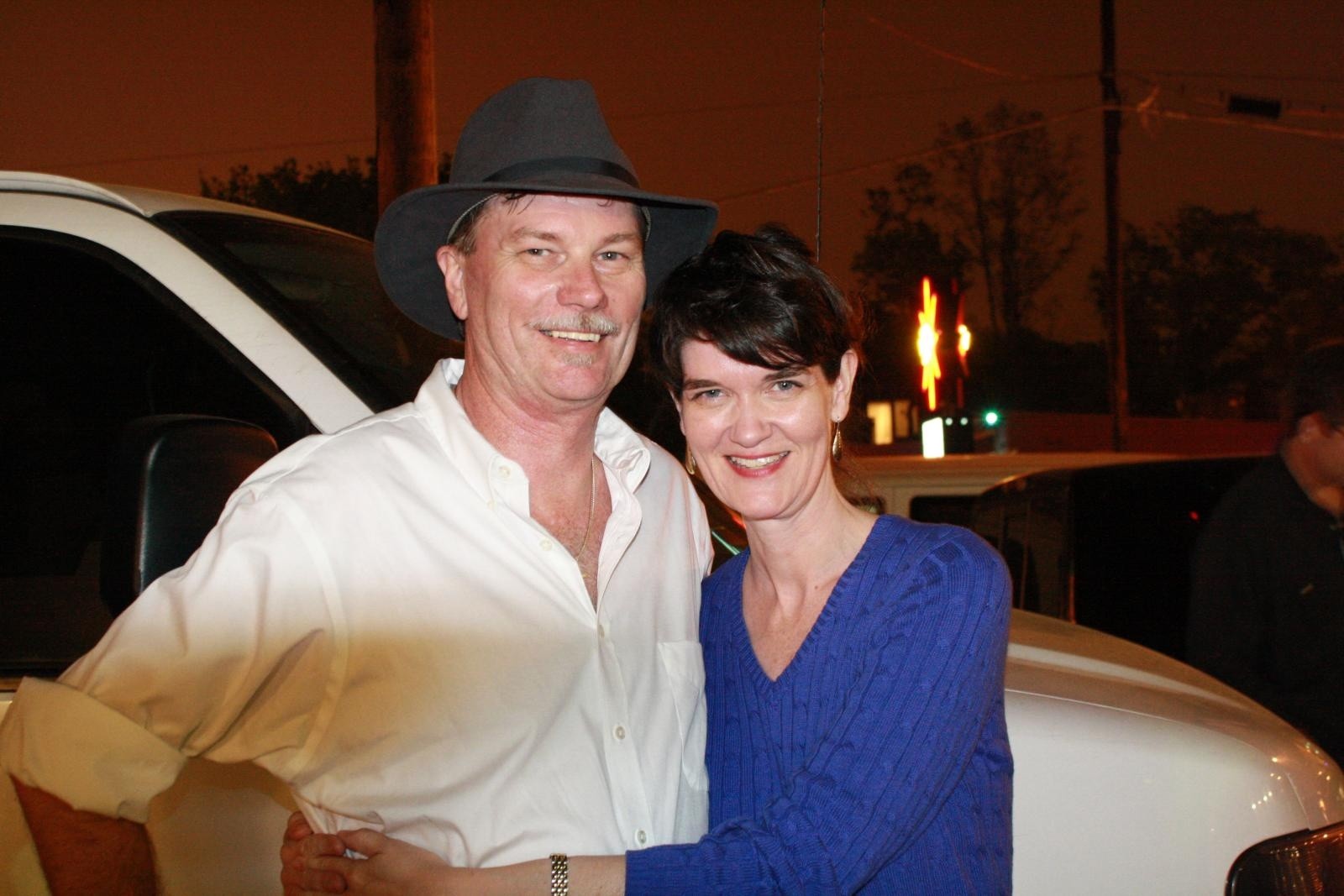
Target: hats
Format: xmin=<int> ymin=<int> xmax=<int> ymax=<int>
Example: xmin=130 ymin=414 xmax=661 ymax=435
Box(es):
xmin=374 ymin=76 xmax=721 ymax=343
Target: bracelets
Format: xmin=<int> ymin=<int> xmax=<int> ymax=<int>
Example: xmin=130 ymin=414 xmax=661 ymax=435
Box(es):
xmin=550 ymin=853 xmax=568 ymax=896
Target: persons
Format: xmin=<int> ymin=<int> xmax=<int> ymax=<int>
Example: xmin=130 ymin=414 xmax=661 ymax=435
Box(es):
xmin=1183 ymin=338 xmax=1344 ymax=761
xmin=0 ymin=79 xmax=721 ymax=896
xmin=280 ymin=230 xmax=1013 ymax=896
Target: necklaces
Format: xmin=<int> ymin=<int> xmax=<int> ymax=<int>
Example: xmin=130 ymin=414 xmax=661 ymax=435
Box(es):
xmin=574 ymin=457 xmax=595 ymax=561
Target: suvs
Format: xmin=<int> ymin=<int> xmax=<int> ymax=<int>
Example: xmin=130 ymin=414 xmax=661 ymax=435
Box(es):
xmin=0 ymin=169 xmax=1343 ymax=896
